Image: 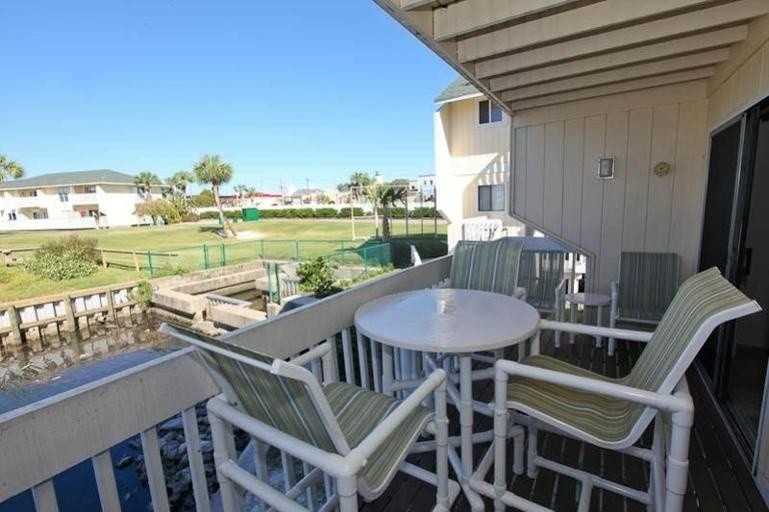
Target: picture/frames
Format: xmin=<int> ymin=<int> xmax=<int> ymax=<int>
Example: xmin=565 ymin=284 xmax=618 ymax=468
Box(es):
xmin=597 ymin=156 xmax=615 ymax=180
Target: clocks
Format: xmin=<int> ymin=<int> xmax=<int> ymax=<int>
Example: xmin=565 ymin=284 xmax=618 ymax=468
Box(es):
xmin=654 ymin=159 xmax=671 ymax=178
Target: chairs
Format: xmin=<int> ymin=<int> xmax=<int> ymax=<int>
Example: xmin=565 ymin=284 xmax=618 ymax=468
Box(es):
xmin=513 ymin=248 xmax=565 ymax=349
xmin=158 ymin=319 xmax=461 ymax=512
xmin=470 ymin=265 xmax=764 ymax=512
xmin=608 ymin=250 xmax=682 ymax=356
xmin=432 ymin=236 xmax=528 ymax=398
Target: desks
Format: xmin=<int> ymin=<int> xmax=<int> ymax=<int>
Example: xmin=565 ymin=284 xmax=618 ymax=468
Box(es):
xmin=354 ymin=286 xmax=540 ymax=510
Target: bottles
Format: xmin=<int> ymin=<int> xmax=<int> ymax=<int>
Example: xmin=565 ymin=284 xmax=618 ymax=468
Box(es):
xmin=437 ymin=289 xmax=456 ymax=316
